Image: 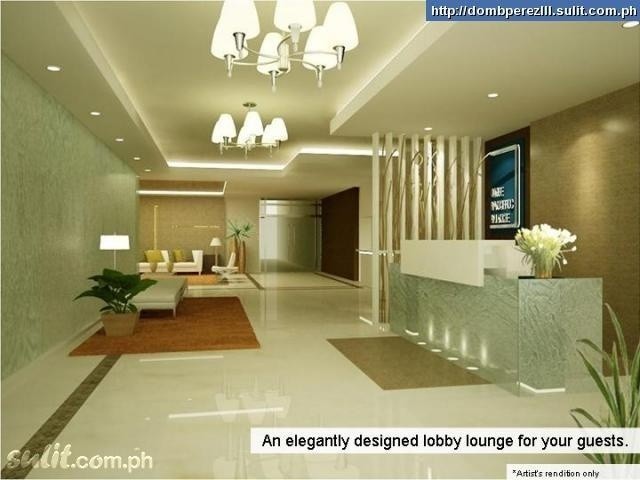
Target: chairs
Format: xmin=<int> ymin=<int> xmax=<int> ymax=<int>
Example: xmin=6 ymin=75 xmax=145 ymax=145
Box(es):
xmin=173 ymin=249 xmax=204 ymax=278
xmin=211 ymin=251 xmax=236 ymax=282
xmin=137 ymin=249 xmax=171 ymax=273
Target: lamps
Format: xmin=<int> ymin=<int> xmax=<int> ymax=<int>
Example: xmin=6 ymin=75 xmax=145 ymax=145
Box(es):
xmin=209 ymin=1 xmax=359 ymax=92
xmin=210 ymin=101 xmax=289 ymax=158
xmin=209 ymin=237 xmax=222 ymax=266
xmin=100 ymin=230 xmax=131 ymax=271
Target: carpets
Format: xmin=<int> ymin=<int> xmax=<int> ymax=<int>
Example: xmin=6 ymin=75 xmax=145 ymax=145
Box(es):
xmin=138 ymin=271 xmax=231 ymax=286
xmin=324 ymin=334 xmax=492 ymax=390
xmin=67 ymin=296 xmax=263 ymax=357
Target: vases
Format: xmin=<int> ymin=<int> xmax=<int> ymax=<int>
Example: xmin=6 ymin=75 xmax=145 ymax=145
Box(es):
xmin=533 ymin=259 xmax=553 ymax=279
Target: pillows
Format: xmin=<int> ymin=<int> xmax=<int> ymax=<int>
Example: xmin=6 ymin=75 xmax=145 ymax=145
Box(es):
xmin=180 ymin=249 xmax=193 ymax=262
xmin=172 ymin=248 xmax=181 ymax=262
xmin=143 ymin=249 xmax=164 ymax=263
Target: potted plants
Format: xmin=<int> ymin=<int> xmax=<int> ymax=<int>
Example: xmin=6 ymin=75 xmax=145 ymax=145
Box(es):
xmin=72 ymin=269 xmax=160 ymax=339
xmin=239 ymin=221 xmax=257 ymax=275
xmin=223 ymin=217 xmax=241 ymax=267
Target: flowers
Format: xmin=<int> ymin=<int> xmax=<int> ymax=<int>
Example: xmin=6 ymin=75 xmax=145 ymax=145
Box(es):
xmin=514 ymin=223 xmax=579 ymax=279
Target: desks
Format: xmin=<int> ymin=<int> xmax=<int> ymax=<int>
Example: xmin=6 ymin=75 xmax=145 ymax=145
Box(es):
xmin=216 ymin=266 xmax=239 ymax=282
